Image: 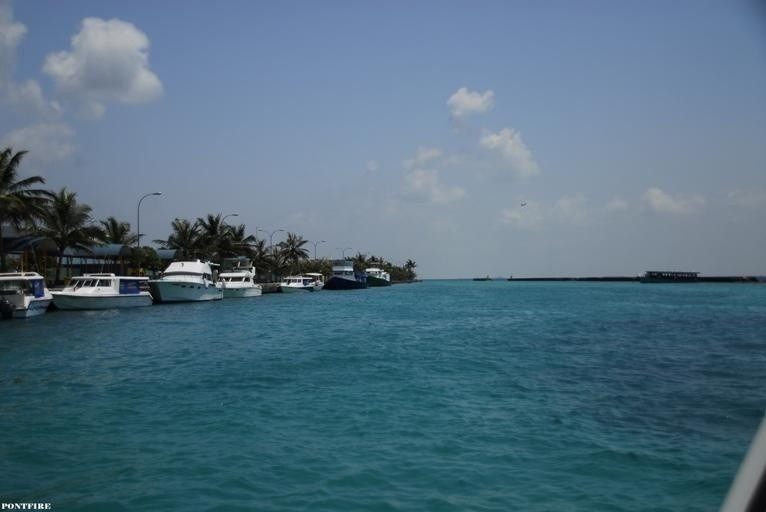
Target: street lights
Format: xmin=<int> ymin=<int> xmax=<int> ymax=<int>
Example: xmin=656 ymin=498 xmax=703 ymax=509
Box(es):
xmin=219 ymin=213 xmax=239 ymax=226
xmin=308 ymin=240 xmax=326 ymax=260
xmin=258 ymin=229 xmax=285 ymax=255
xmin=138 ymin=192 xmax=162 ymax=247
xmin=336 ymin=247 xmax=352 ymax=258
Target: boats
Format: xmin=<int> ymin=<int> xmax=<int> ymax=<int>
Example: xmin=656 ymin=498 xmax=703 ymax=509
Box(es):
xmin=51 ymin=273 xmax=153 ymax=309
xmin=148 ymin=260 xmax=224 ymax=301
xmin=640 ymin=271 xmax=700 ymax=283
xmin=324 ymin=266 xmax=367 ymax=289
xmin=216 ymin=262 xmax=262 ymax=297
xmin=0 ymin=271 xmax=52 ymax=317
xmin=281 ymin=272 xmax=325 ymax=293
xmin=364 ymin=268 xmax=392 ymax=286
